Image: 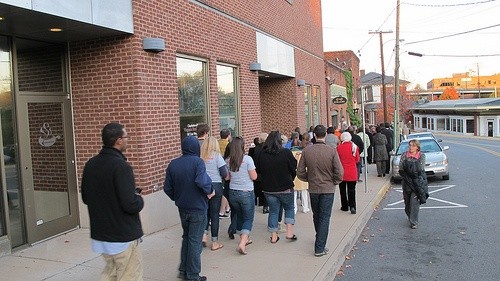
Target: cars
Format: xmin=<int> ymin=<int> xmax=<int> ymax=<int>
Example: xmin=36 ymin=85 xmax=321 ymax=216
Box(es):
xmin=408 ymin=132 xmax=443 ymax=143
xmin=391 ymin=137 xmax=449 ymax=181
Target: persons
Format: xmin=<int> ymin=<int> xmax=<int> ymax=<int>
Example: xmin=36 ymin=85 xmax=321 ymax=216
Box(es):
xmin=399 ymin=140 xmax=425 ymax=228
xmin=164 ymin=136 xmax=215 ymax=281
xmin=197 ymin=124 xmax=210 ymax=146
xmin=249 ymin=133 xmax=286 ymax=233
xmin=81 ymin=123 xmax=144 ymax=281
xmin=201 ymin=136 xmax=230 ymax=250
xmin=336 ymin=132 xmax=360 ymax=213
xmin=400 ymin=123 xmax=410 ymax=142
xmin=340 ymin=123 xmax=393 ymax=181
xmin=224 ymin=137 xmax=258 ymax=254
xmin=258 ymin=131 xmax=297 ymax=243
xmin=218 ymin=129 xmax=232 ymax=218
xmin=287 ymin=126 xmax=315 ymax=215
xmin=297 ymin=125 xmax=344 ymax=255
xmin=326 ymin=126 xmax=341 ymax=148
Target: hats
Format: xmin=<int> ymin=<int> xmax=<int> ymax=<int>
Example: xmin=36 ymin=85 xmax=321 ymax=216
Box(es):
xmin=340 ymin=132 xmax=352 ymax=142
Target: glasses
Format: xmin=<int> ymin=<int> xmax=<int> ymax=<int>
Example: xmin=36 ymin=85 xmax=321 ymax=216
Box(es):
xmin=117 ymin=135 xmax=131 ymax=141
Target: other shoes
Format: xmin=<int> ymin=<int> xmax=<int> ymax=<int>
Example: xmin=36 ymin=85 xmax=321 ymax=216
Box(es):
xmin=199 ymin=276 xmax=206 ymax=281
xmin=340 ymin=206 xmax=348 ymax=211
xmin=277 ymin=229 xmax=286 ymax=233
xmin=228 ymin=228 xmax=235 ymax=239
xmin=377 ymin=172 xmax=385 ymax=177
xmin=218 ymin=213 xmax=228 ymax=218
xmin=358 ymin=179 xmax=363 ymax=182
xmin=314 ymin=248 xmax=329 ymax=256
xmin=178 ymin=273 xmax=186 ymax=279
xmin=411 ymin=224 xmax=417 ymax=229
xmin=350 ymin=207 xmax=356 ymax=214
xmin=263 ymin=210 xmax=270 ymax=214
xmin=225 ymin=208 xmax=231 ymax=216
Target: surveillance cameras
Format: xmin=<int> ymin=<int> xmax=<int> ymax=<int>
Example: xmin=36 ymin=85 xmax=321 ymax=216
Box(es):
xmin=325 ymin=76 xmax=329 ymax=80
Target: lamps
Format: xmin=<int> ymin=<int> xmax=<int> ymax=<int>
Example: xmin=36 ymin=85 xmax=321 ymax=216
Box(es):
xmin=142 ymin=37 xmax=165 ymax=53
xmin=249 ymin=63 xmax=261 ymax=71
xmin=298 ymin=80 xmax=306 ymax=86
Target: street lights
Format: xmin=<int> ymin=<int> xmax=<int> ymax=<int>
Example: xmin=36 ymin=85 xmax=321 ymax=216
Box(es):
xmin=357 ymin=84 xmax=372 ymax=192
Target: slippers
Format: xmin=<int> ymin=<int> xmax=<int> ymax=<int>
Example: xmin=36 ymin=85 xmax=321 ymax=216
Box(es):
xmin=202 ymin=241 xmax=207 ymax=247
xmin=270 ymin=236 xmax=280 ymax=243
xmin=236 ymin=247 xmax=247 ymax=255
xmin=245 ymin=239 xmax=252 ymax=246
xmin=285 ymin=235 xmax=297 ymax=240
xmin=211 ymin=243 xmax=224 ymax=250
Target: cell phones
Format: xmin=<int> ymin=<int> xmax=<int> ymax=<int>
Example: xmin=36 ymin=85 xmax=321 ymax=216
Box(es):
xmin=135 ymin=188 xmax=142 ymax=193
